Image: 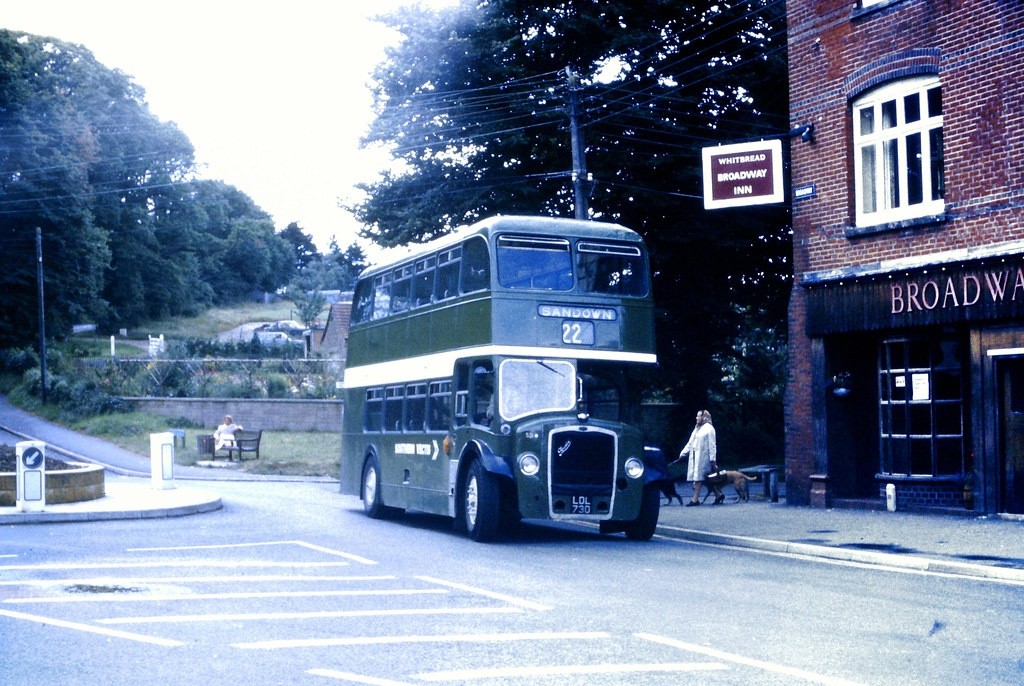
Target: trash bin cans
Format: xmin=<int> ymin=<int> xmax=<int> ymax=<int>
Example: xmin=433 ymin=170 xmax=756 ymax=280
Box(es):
xmin=809 ymin=475 xmax=830 ymax=509
xmin=197 ymin=435 xmax=215 ymax=454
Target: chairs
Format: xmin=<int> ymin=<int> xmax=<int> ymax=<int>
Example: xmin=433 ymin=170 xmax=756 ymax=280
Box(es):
xmin=416 ymin=289 xmax=448 ymax=306
xmin=395 ymin=419 xmax=426 ymax=431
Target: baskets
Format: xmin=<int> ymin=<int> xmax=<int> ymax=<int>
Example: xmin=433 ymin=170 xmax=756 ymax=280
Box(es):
xmin=705 ymin=464 xmax=728 ymax=485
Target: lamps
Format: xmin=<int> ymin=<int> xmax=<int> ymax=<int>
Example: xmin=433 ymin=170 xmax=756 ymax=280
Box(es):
xmin=802 ymin=125 xmax=812 ymax=144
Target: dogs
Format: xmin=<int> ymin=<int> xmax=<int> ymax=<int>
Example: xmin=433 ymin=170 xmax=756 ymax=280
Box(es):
xmin=659 ymin=474 xmax=686 ymax=506
xmin=701 ymin=469 xmax=757 ymax=506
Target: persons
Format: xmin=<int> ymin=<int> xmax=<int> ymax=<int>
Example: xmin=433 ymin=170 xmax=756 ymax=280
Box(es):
xmin=680 ymin=409 xmax=725 ymax=506
xmin=214 ymin=414 xmax=243 ymax=451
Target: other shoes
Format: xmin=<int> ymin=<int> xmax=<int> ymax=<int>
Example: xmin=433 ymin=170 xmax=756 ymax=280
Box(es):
xmin=687 ymin=500 xmax=700 ymax=506
xmin=712 ymin=494 xmax=724 ymax=505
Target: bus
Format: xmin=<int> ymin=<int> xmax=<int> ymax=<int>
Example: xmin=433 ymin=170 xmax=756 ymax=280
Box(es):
xmin=337 ymin=213 xmax=676 ymax=543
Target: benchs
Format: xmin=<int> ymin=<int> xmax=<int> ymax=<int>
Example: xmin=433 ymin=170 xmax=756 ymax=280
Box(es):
xmin=209 ymin=425 xmax=264 ymax=462
